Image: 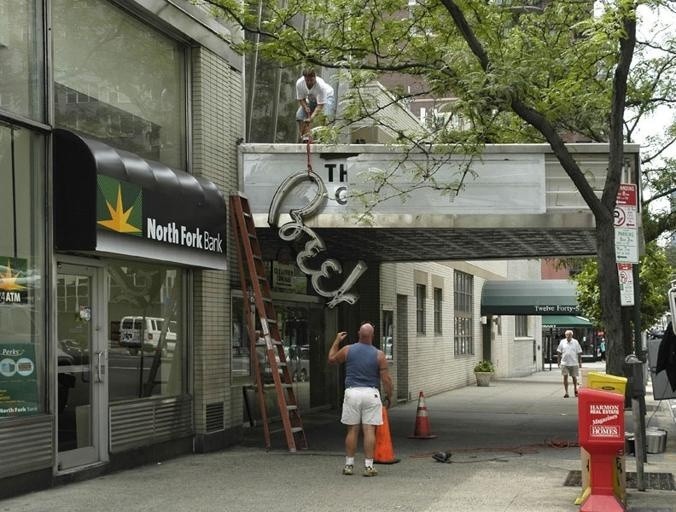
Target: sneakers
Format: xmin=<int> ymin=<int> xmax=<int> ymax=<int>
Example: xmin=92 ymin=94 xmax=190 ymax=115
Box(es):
xmin=363 ymin=465 xmax=378 ymax=477
xmin=342 ymin=465 xmax=353 ymax=475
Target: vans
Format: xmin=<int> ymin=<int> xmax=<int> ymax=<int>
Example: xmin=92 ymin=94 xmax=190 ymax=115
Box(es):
xmin=0 ymin=265 xmax=76 ymax=414
xmin=119 ymin=316 xmax=177 ymax=358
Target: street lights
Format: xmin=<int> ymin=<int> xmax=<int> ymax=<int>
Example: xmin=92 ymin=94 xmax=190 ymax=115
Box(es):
xmin=583 ymin=337 xmax=586 ymax=355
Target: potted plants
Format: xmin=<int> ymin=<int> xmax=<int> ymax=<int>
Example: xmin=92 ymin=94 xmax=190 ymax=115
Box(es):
xmin=472 ymin=359 xmax=495 ymax=387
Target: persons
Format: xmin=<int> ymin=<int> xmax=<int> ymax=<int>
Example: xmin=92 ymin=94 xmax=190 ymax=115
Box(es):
xmin=294 ymin=66 xmax=336 ymax=143
xmin=327 ymin=322 xmax=392 ymax=477
xmin=600 ymin=338 xmax=605 ymax=362
xmin=556 ymin=330 xmax=583 ymax=398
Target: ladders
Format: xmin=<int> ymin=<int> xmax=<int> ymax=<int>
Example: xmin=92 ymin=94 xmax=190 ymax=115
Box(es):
xmin=146 ymin=279 xmax=180 ymax=396
xmin=229 ymin=195 xmax=309 ymax=453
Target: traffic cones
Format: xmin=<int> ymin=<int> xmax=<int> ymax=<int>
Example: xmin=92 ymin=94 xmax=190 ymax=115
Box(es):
xmin=407 ymin=391 xmax=438 ymax=439
xmin=373 ymin=407 xmax=400 ymax=464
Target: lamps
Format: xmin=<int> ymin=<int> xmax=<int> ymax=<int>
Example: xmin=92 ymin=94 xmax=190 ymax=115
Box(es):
xmin=492 ymin=317 xmax=501 ymax=325
xmin=480 ymin=316 xmax=487 ymax=324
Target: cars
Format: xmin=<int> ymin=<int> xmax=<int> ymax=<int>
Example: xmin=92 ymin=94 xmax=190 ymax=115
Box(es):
xmin=233 ymin=323 xmax=309 ymax=383
xmin=383 ymin=336 xmax=393 ymax=359
